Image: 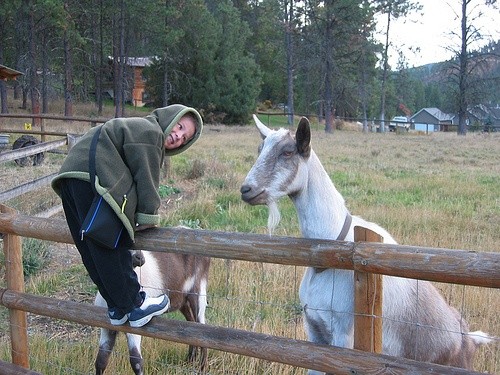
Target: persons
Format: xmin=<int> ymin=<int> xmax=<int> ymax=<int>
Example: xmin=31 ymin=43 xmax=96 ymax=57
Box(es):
xmin=51 ymin=104 xmax=203 ymax=328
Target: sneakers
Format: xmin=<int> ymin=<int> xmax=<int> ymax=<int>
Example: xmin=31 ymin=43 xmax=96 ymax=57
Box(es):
xmin=107 ymin=294 xmax=170 ymax=328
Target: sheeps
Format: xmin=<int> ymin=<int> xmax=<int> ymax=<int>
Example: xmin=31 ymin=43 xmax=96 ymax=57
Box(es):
xmin=238 ymin=113 xmax=496 ymax=375
xmin=93 ymin=224 xmax=212 ymax=375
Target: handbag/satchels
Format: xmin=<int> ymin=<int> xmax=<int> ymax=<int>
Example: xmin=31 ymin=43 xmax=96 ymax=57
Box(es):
xmin=79 ymin=194 xmax=124 ymax=250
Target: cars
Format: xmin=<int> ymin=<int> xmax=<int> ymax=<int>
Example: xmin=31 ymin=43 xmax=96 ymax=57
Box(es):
xmin=390 ymin=115 xmax=410 ymax=131
